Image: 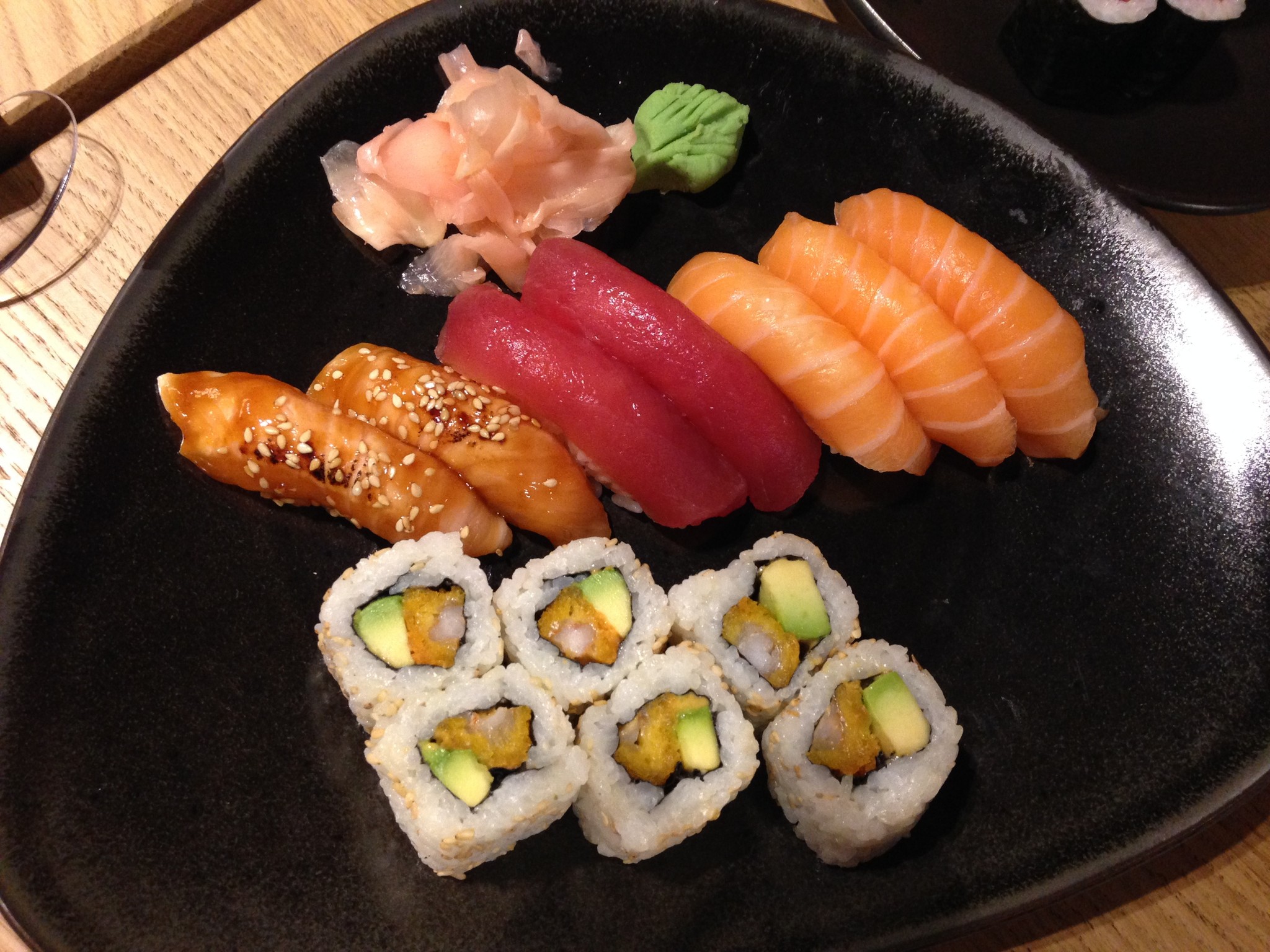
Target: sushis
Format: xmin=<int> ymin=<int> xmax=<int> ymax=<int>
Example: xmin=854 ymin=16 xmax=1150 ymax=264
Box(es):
xmin=361 ymin=662 xmax=589 ymax=879
xmin=430 ymin=239 xmax=826 ymax=528
xmin=314 ymin=529 xmax=505 ymax=729
xmin=759 ymin=639 xmax=963 ymax=865
xmin=157 ymin=344 xmax=611 ymax=559
xmin=573 ymin=641 xmax=764 ymax=865
xmin=664 ymin=533 xmax=858 ymax=732
xmin=489 ymin=535 xmax=673 ymax=711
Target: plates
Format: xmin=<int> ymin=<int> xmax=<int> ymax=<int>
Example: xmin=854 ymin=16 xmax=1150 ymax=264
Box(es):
xmin=832 ymin=3 xmax=1269 ymax=218
xmin=5 ymin=1 xmax=1264 ymax=952
